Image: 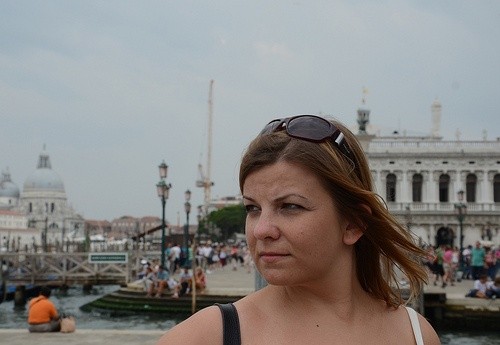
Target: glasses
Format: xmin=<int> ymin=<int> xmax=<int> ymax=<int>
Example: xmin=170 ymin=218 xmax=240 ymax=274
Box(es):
xmin=260 ymin=115 xmax=362 ymax=184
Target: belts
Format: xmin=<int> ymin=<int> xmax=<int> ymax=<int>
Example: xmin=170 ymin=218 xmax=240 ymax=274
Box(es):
xmin=29 ymin=323 xmax=42 ymax=325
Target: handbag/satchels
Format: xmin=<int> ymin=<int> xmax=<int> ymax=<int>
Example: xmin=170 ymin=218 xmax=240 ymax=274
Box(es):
xmin=59 ymin=312 xmax=75 ymax=333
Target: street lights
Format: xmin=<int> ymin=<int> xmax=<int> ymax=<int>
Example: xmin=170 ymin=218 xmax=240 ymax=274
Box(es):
xmin=454 ymin=188 xmax=467 ymax=268
xmin=156 ymin=158 xmax=172 ymax=266
xmin=184 ymin=188 xmax=191 ymax=265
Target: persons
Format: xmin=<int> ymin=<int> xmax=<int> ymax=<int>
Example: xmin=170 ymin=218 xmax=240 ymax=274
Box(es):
xmin=156 ymin=115 xmax=443 ymax=345
xmin=140 ymin=242 xmax=256 ymax=300
xmin=420 ymin=239 xmax=500 ymax=300
xmin=28 ymin=289 xmax=65 ymax=333
